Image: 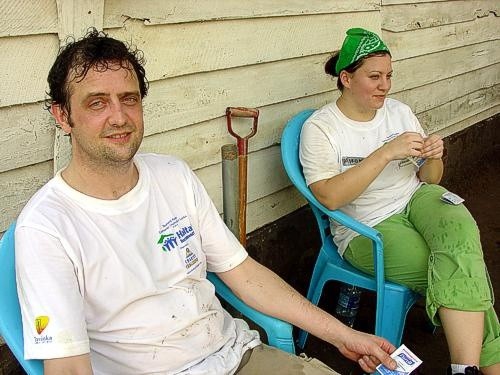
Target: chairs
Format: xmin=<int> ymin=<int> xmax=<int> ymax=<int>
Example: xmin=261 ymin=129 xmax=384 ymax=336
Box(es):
xmin=0 ymin=219 xmax=295 ymax=375
xmin=281 ymin=109 xmax=422 ymax=349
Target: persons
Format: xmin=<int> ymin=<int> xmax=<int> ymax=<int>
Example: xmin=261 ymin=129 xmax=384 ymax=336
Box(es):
xmin=299 ymin=26 xmax=500 ymax=375
xmin=13 ymin=35 xmax=396 ymax=375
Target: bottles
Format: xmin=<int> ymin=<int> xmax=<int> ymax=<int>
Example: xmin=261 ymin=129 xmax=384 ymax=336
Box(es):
xmin=335 ymin=283 xmax=361 ymax=328
